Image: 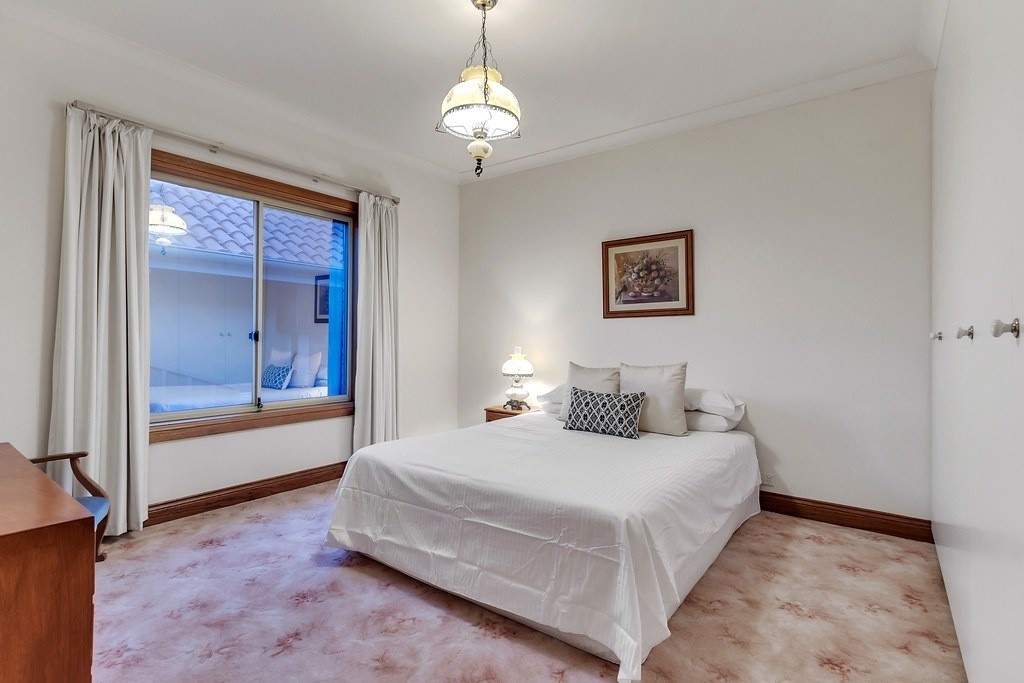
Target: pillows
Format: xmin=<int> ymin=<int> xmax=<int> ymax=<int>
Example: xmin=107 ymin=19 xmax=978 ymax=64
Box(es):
xmin=289 ymin=351 xmax=322 ymax=387
xmin=619 ymin=362 xmax=690 ymax=436
xmin=316 ymin=367 xmax=329 ymax=380
xmin=563 ymin=387 xmax=647 ymax=440
xmin=268 ymin=347 xmax=297 ymax=369
xmin=314 ymin=379 xmax=328 ymax=386
xmin=261 ymin=364 xmax=294 ymax=390
xmin=542 ymin=402 xmax=738 ymax=431
xmin=537 ymin=382 xmax=747 ymax=422
xmin=557 ymin=360 xmax=621 ymax=421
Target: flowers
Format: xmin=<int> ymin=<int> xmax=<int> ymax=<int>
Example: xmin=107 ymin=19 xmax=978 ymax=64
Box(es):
xmin=620 ymin=251 xmax=676 ymax=297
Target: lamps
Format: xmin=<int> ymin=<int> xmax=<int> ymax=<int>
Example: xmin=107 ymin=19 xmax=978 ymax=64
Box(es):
xmin=501 ymin=346 xmax=535 ymax=411
xmin=148 ymin=182 xmax=188 ymax=255
xmin=433 ymin=0 xmax=521 ymax=179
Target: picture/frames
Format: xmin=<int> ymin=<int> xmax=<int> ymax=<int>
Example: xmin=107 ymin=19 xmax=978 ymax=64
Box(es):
xmin=313 ymin=274 xmax=330 ymax=324
xmin=602 ymin=228 xmax=695 ymax=318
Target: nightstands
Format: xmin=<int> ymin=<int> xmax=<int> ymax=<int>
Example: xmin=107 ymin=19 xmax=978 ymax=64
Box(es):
xmin=484 ymin=406 xmax=539 ymax=422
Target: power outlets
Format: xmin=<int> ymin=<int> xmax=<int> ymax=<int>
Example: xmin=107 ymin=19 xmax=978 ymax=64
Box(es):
xmin=762 ymin=473 xmax=775 ymax=486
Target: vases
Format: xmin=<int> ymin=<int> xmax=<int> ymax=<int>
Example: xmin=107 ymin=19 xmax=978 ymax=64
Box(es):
xmin=634 ymin=283 xmax=660 ymax=296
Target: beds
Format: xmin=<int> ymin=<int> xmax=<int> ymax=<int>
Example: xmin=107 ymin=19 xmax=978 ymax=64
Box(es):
xmin=150 ymin=383 xmax=328 ymax=412
xmin=324 ymin=410 xmax=762 ymax=683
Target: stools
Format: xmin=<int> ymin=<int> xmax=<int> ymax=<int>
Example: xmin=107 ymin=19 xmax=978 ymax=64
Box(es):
xmin=28 ymin=451 xmax=111 ymax=562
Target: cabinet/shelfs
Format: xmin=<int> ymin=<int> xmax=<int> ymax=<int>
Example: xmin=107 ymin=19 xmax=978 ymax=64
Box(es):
xmin=1 ymin=442 xmax=97 ymax=683
